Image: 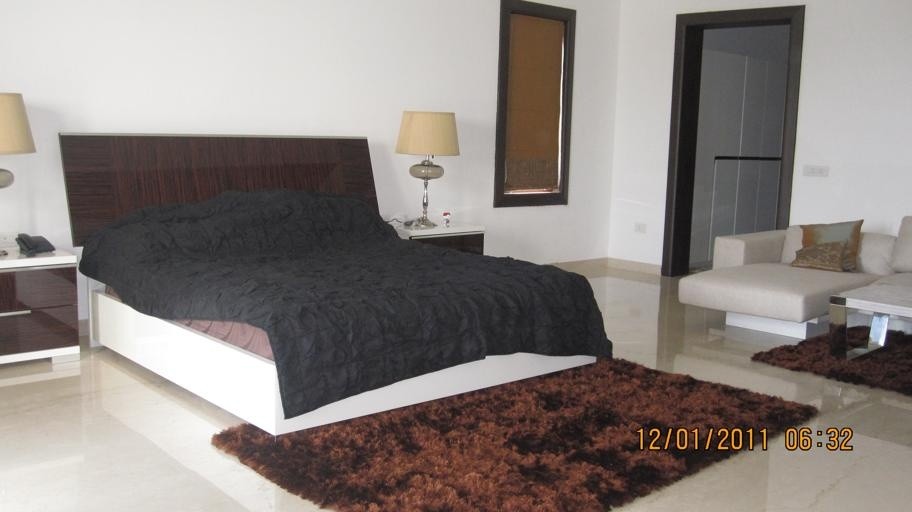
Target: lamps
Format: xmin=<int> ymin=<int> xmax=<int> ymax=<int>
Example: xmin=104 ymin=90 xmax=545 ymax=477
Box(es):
xmin=396 ymin=111 xmax=459 ymax=229
xmin=0 ymin=93 xmax=36 ymax=188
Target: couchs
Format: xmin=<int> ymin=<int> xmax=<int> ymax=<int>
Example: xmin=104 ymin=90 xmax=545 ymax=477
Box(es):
xmin=678 ymin=229 xmax=898 ymax=340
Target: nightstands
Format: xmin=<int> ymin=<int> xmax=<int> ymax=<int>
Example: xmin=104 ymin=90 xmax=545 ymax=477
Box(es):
xmin=0 ymin=246 xmax=81 ymax=365
xmin=389 ymin=221 xmax=486 ymax=257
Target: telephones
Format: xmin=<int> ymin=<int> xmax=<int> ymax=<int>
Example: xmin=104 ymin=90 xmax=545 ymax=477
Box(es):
xmin=16 ymin=233 xmax=55 ymax=255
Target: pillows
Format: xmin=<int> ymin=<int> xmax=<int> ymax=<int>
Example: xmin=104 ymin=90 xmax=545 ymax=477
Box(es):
xmin=791 ymin=239 xmax=847 ymax=273
xmin=780 ymin=224 xmax=897 ymax=277
xmin=799 ymin=219 xmax=864 ymax=271
xmin=892 ymin=216 xmax=912 ymax=272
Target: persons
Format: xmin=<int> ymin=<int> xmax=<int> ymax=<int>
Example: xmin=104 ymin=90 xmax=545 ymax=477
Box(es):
xmin=442 ymin=212 xmax=451 ymax=228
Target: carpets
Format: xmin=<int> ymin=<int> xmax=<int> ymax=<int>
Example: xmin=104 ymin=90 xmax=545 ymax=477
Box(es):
xmin=211 ymin=354 xmax=818 ymax=511
xmin=751 ymin=326 xmax=912 ymax=396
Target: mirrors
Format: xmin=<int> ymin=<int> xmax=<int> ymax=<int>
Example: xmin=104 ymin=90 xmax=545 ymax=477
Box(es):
xmin=492 ymin=1 xmax=576 ymax=207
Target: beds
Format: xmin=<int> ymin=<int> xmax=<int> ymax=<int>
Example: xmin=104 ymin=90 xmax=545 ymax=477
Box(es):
xmin=57 ymin=133 xmax=612 ymax=443
